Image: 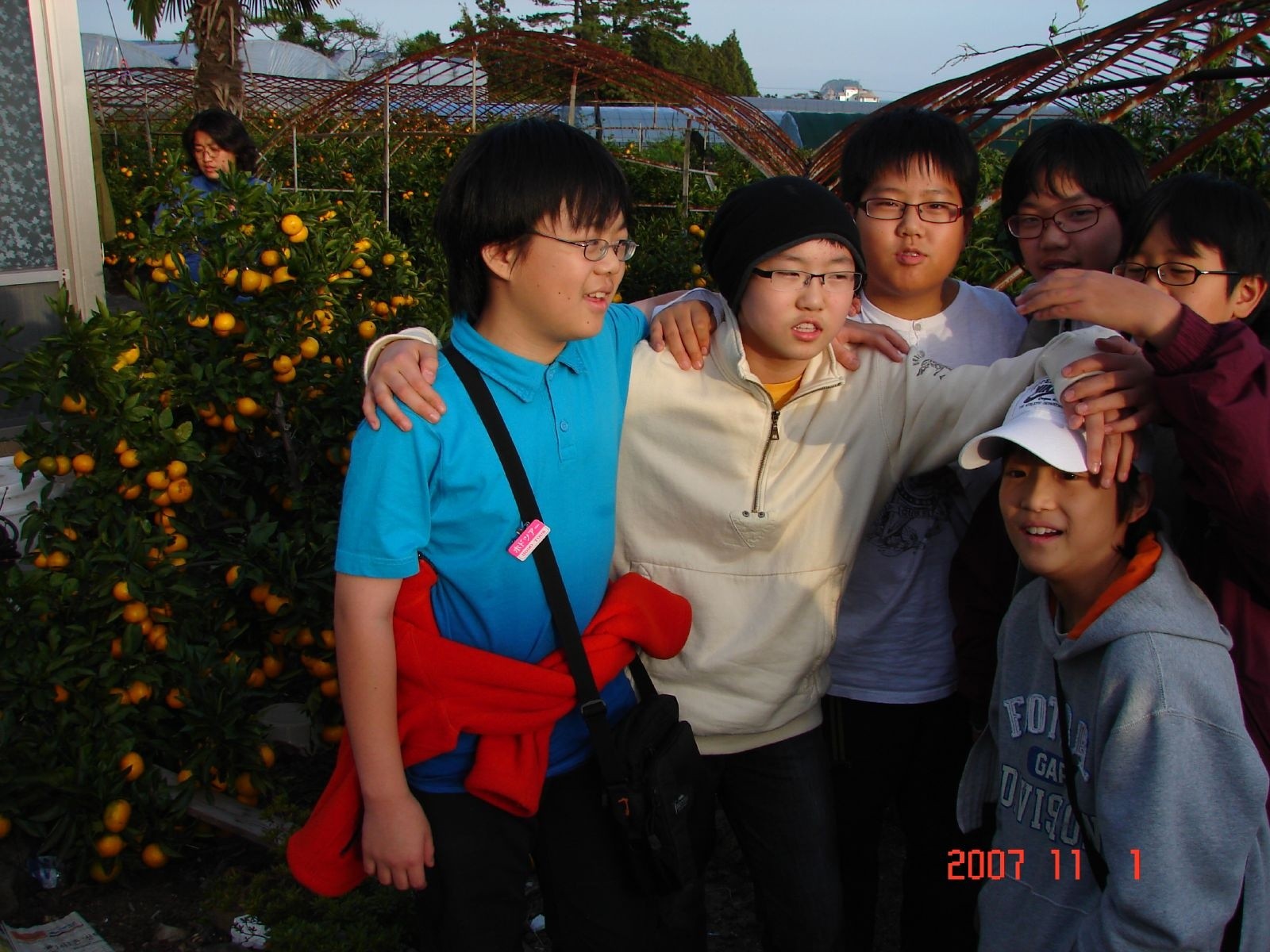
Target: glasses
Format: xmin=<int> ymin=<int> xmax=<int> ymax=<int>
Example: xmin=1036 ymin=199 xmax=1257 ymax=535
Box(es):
xmin=753 ymin=267 xmax=864 ymax=295
xmin=192 ymin=148 xmax=221 ymax=160
xmin=1112 ymin=262 xmax=1240 ymax=286
xmin=858 ymin=197 xmax=965 ymax=224
xmin=1005 ymin=202 xmax=1112 ymax=239
xmin=533 ymin=229 xmax=639 ymax=262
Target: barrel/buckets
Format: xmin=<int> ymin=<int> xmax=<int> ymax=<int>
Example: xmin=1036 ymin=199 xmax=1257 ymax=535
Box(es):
xmin=257 ymin=702 xmax=312 ymax=758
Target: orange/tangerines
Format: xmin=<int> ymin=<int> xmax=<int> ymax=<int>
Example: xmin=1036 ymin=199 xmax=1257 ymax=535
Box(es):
xmin=0 ymin=101 xmax=709 ymax=882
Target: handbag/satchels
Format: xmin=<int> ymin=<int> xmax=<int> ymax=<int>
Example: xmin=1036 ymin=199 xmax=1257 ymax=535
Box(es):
xmin=603 ymin=690 xmax=720 ymax=892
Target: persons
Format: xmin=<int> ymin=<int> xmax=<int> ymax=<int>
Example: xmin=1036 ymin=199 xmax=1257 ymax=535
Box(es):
xmin=1016 ymin=175 xmax=1270 ymax=492
xmin=690 ymin=128 xmax=707 ymax=161
xmin=649 ymin=108 xmax=1028 ymax=952
xmin=151 ymin=108 xmax=273 ymax=302
xmin=288 ymin=115 xmax=910 ymax=952
xmin=1000 ymin=118 xmax=1167 ymax=435
xmin=957 ymin=375 xmax=1270 ymax=952
xmin=363 ymin=174 xmax=1134 ymax=952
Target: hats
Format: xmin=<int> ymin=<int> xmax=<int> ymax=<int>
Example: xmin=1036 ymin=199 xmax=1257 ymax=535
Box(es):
xmin=958 ymin=375 xmax=1088 ymax=472
xmin=701 ymin=176 xmax=866 ymax=314
